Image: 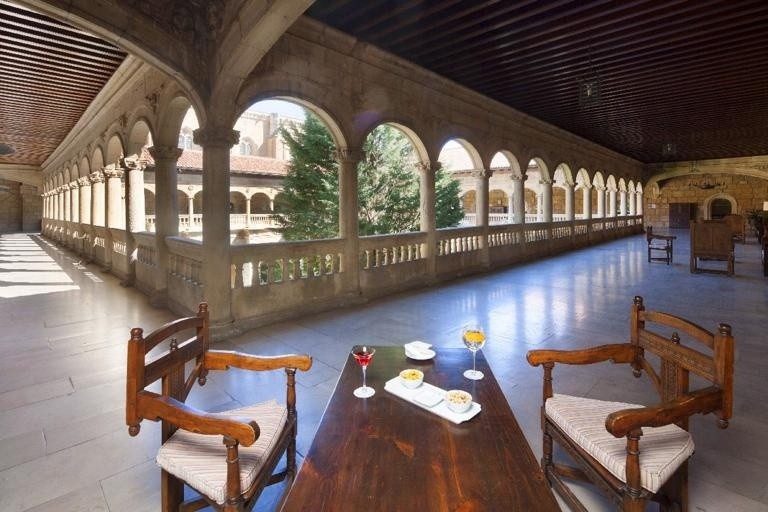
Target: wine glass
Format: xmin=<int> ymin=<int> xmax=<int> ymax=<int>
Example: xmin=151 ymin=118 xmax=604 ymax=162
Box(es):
xmin=463 ymin=324 xmax=485 ymax=381
xmin=350 ymin=345 xmax=377 ymax=398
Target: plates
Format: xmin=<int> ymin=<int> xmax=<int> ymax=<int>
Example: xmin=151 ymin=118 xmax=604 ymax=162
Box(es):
xmin=404 ymin=349 xmax=436 ymax=361
xmin=383 ymin=375 xmax=482 ymax=424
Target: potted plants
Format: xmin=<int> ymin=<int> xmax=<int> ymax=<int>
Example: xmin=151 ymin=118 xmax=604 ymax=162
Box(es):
xmin=746 ymin=209 xmax=768 ymax=245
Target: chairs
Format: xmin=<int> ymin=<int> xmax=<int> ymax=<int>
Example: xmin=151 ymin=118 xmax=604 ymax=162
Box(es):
xmin=525 ymin=296 xmax=734 ymax=512
xmin=647 ymin=225 xmax=677 ymax=265
xmin=722 ymin=214 xmax=745 ymax=245
xmin=690 ymin=223 xmax=736 ymax=277
xmin=126 ymin=302 xmax=312 ymax=512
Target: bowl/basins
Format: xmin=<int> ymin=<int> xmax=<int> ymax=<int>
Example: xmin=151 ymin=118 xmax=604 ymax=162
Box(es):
xmin=405 ymin=341 xmax=432 ymax=355
xmin=446 ymin=389 xmax=472 ymax=413
xmin=399 ymin=369 xmax=424 ymax=389
